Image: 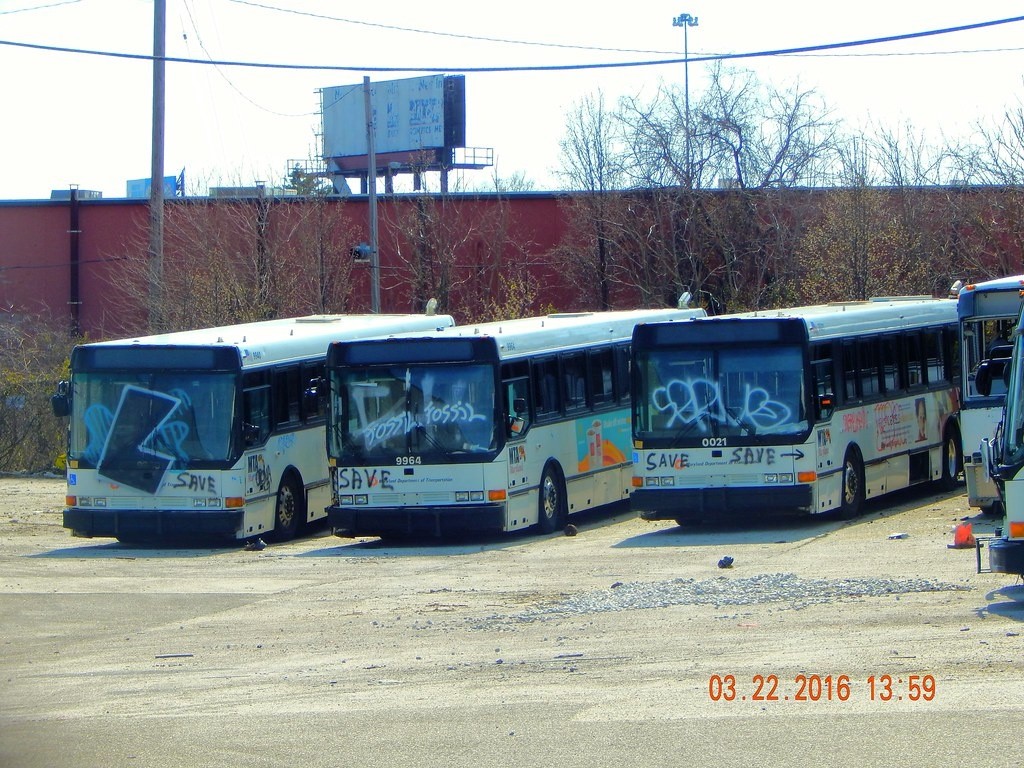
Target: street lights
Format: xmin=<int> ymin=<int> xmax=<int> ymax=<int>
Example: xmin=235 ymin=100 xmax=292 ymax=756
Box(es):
xmin=674 ymin=13 xmax=700 ymax=189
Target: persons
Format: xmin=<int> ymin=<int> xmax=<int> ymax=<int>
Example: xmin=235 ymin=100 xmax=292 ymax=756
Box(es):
xmin=914 ymin=397 xmax=928 ymax=443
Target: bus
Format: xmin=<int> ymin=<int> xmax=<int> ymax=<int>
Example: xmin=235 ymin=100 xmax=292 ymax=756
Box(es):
xmin=51 ymin=299 xmax=456 ymax=546
xmin=628 ymin=281 xmax=970 ymax=528
xmin=307 ymin=292 xmax=709 ymax=543
xmin=955 ymin=274 xmax=1024 ymax=514
xmin=975 ymin=289 xmax=1024 ymax=580
